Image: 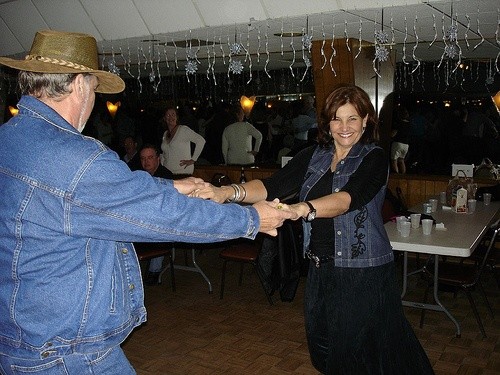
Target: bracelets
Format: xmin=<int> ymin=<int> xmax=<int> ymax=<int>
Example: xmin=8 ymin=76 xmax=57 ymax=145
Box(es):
xmin=226 ymin=184 xmax=247 ymax=204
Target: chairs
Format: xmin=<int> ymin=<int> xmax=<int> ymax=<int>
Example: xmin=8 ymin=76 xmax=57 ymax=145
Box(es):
xmin=219 ymin=226 xmax=274 ymax=306
xmin=418 ymin=224 xmax=500 ymax=338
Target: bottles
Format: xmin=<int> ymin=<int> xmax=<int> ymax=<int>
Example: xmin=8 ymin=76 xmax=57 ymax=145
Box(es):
xmin=239 ymin=167 xmax=247 ymax=184
xmin=455 ymin=186 xmax=468 ymax=214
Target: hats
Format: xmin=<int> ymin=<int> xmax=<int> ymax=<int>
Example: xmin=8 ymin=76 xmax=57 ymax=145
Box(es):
xmin=0 ymin=31 xmax=125 ymax=94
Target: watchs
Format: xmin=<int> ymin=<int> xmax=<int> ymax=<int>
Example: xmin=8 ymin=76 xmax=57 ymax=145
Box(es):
xmin=301 ymin=201 xmax=316 ymax=223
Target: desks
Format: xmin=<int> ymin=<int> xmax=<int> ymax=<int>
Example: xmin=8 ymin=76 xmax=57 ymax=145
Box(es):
xmin=194 ymin=166 xmax=274 ymax=184
xmin=383 ymin=194 xmax=500 ymax=339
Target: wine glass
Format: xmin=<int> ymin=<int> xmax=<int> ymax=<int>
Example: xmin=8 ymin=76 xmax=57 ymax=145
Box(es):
xmin=468 ymin=183 xmax=478 ymax=198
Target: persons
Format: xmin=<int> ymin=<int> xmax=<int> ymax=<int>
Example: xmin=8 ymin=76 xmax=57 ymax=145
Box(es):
xmin=269 ymin=95 xmax=317 ymax=165
xmin=140 ymin=146 xmax=173 ymax=284
xmin=219 ymin=109 xmax=263 ymax=168
xmin=391 ymin=109 xmax=412 ymax=174
xmin=188 ymin=87 xmax=437 ymax=375
xmin=0 ymin=29 xmax=299 ymax=375
xmin=121 ymin=137 xmax=142 ymax=170
xmin=160 ymin=109 xmax=205 ymax=179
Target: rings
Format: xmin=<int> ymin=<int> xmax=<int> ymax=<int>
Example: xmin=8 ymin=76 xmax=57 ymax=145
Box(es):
xmin=194 ymin=188 xmax=200 ymax=193
xmin=277 ymin=206 xmax=281 ymax=209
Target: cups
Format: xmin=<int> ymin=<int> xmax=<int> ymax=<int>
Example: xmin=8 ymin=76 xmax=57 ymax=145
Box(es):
xmin=396 ymin=216 xmax=407 ymax=232
xmin=400 ymin=221 xmax=411 ymax=238
xmin=468 ymin=199 xmax=477 ymax=212
xmin=410 ymin=213 xmax=421 ymax=229
xmin=422 ymin=219 xmax=433 ymax=235
xmin=423 ymin=203 xmax=432 ymax=216
xmin=484 ymin=193 xmax=491 ymax=206
xmin=440 ymin=191 xmax=446 ymax=203
xmin=429 ymin=199 xmax=438 ymax=212
